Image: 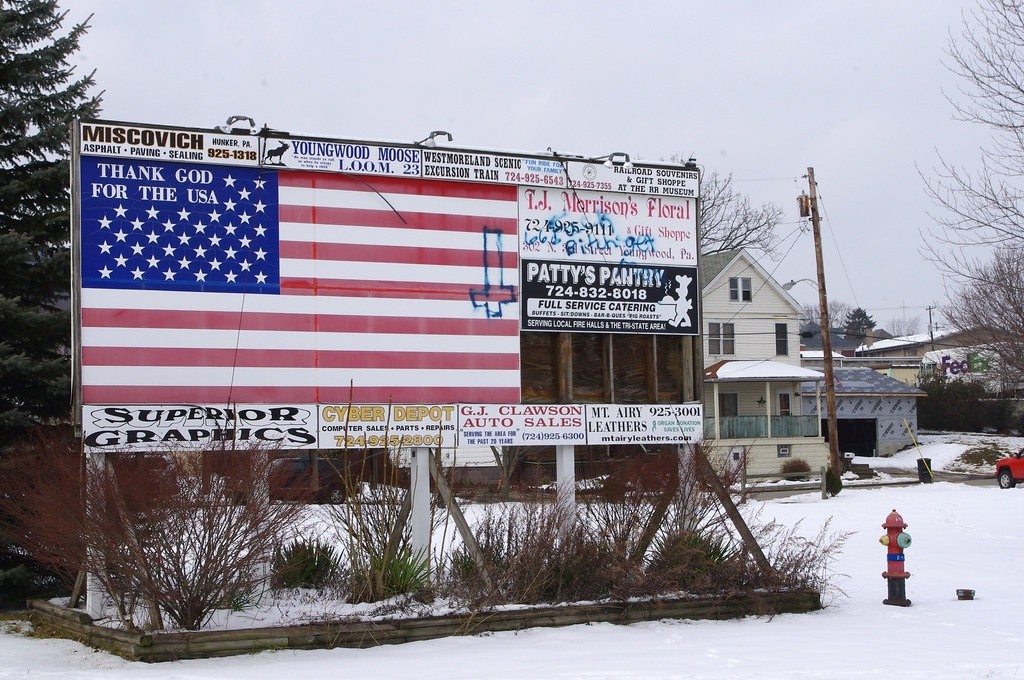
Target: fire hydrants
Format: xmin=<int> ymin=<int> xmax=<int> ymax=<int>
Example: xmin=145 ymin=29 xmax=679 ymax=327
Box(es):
xmin=879 ymin=508 xmax=913 ymax=607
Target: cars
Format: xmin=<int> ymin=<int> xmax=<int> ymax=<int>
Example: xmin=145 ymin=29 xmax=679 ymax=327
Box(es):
xmin=994 ymin=445 xmax=1024 ymax=489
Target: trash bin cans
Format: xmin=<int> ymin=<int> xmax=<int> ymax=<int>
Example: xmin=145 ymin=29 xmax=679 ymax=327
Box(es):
xmin=917 ymin=458 xmax=932 ymax=484
